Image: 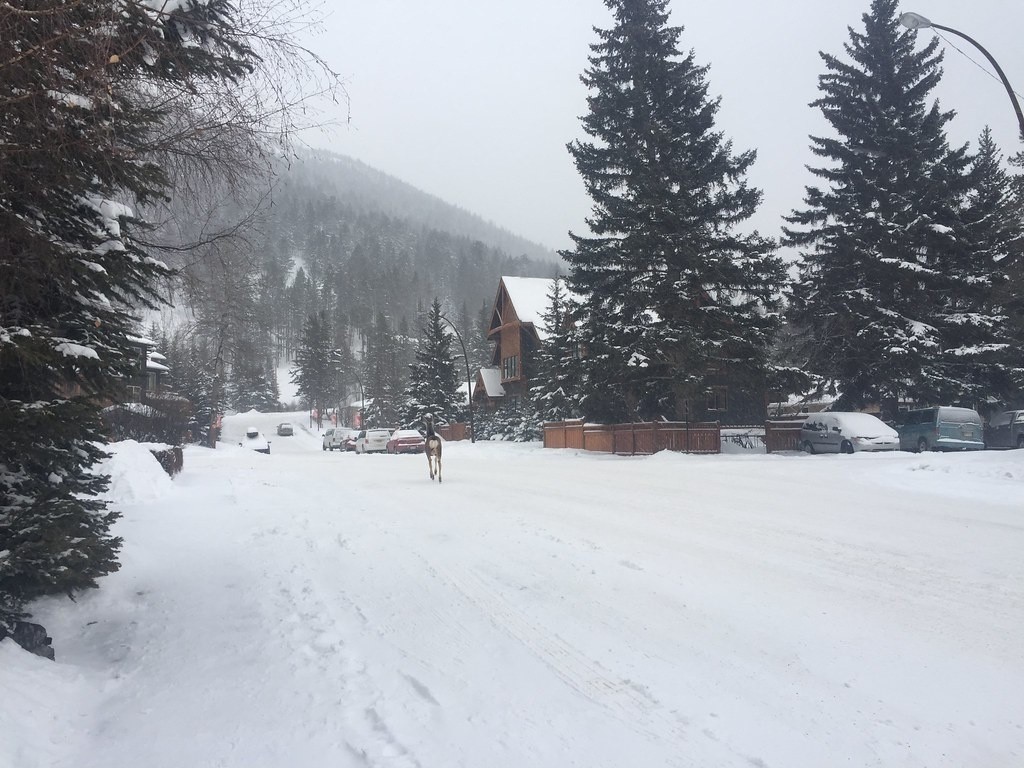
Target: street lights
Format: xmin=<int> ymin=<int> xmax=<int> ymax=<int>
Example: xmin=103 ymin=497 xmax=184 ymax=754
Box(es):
xmin=897 ymin=10 xmax=1024 ymax=139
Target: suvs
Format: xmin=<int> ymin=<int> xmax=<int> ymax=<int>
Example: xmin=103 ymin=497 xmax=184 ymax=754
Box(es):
xmin=239 ymin=432 xmax=271 ymax=453
xmin=322 ymin=428 xmax=391 ymax=454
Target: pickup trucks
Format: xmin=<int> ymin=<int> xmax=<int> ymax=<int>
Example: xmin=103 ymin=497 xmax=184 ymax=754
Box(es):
xmin=983 ymin=410 xmax=1024 ymax=448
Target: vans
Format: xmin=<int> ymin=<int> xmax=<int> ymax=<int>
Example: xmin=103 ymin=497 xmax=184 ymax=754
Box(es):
xmin=893 ymin=406 xmax=984 ymax=454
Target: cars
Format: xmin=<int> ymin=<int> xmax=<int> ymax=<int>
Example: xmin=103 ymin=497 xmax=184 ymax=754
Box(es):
xmin=386 ymin=430 xmax=425 ymax=454
xmin=799 ymin=411 xmax=899 ymax=455
xmin=277 ymin=423 xmax=293 ymax=436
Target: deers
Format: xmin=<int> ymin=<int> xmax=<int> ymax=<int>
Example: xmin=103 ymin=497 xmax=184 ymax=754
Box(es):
xmin=423 ymin=414 xmax=442 ymax=484
